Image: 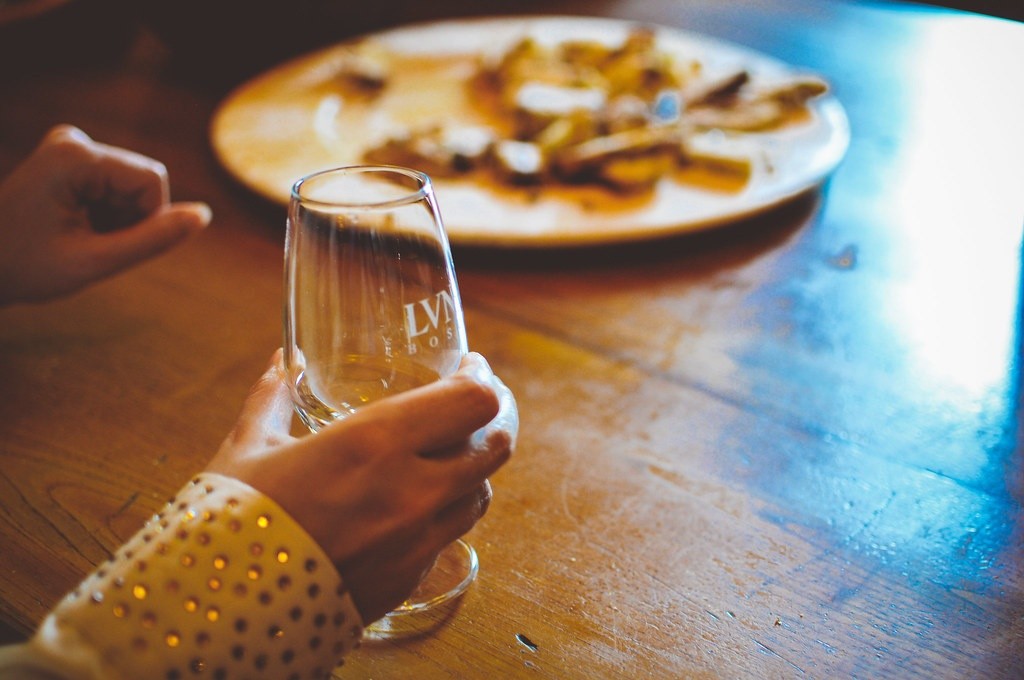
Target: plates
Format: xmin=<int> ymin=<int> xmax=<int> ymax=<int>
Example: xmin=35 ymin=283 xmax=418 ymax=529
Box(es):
xmin=205 ymin=12 xmax=853 ymax=250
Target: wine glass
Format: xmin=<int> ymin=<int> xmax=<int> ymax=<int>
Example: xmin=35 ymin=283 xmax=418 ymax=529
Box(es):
xmin=282 ymin=164 xmax=480 ymax=618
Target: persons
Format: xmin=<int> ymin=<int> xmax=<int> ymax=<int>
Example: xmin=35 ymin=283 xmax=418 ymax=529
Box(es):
xmin=0 ymin=125 xmax=519 ymax=680
